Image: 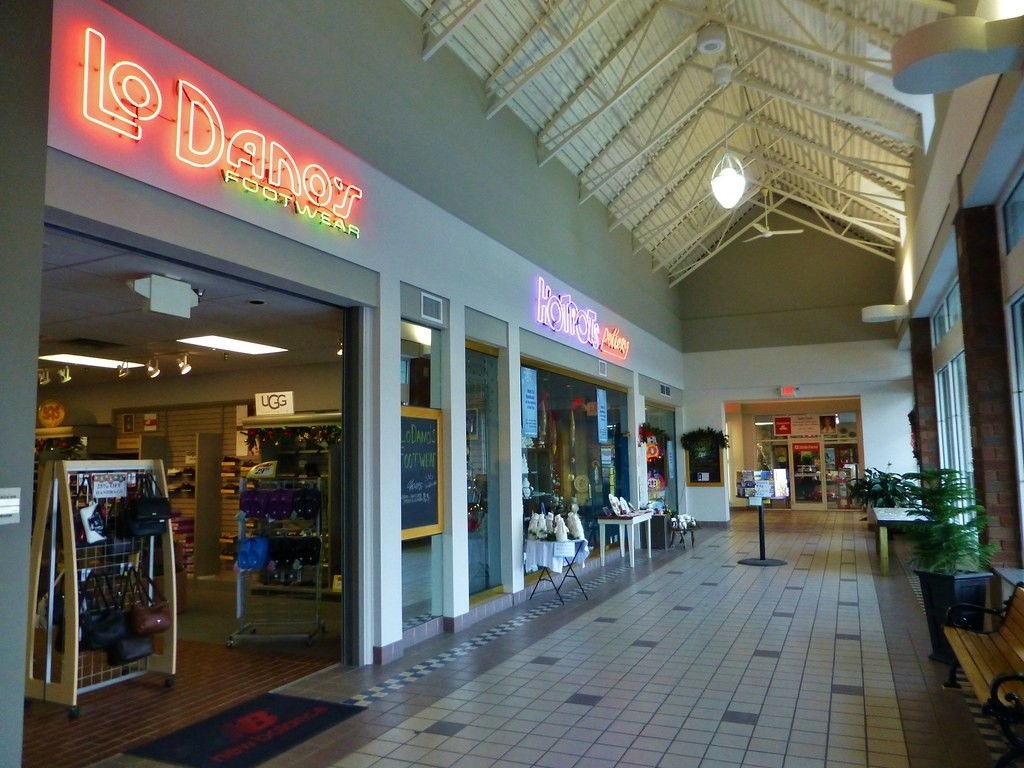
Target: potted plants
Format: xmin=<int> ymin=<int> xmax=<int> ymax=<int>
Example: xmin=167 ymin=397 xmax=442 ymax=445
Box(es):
xmin=846 ymin=468 xmax=915 ymax=540
xmin=903 ymin=469 xmax=993 ymax=665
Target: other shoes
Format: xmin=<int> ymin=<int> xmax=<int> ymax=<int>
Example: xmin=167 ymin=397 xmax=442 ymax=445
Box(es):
xmin=221 ymin=455 xmax=258 ymax=491
xmin=166 ymin=466 xmax=195 ymax=495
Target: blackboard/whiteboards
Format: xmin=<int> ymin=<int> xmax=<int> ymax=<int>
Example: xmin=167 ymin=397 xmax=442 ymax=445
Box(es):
xmin=686 ymin=437 xmax=722 ymax=486
xmin=400 ymin=406 xmax=445 ymax=541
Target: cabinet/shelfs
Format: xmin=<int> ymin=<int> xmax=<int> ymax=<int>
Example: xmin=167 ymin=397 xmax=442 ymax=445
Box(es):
xmin=250 ymin=449 xmax=331 ymax=593
xmin=218 ymin=536 xmax=235 ymax=562
xmin=220 ymin=461 xmax=236 ymax=494
xmin=166 ymin=473 xmax=194 ymax=493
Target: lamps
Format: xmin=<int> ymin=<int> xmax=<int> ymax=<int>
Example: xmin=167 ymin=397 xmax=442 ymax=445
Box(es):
xmin=55 ymin=365 xmax=72 ymax=385
xmin=176 ymin=352 xmax=191 ymax=375
xmin=146 ymin=354 xmax=161 ymax=379
xmin=38 ymin=368 xmax=52 ymax=387
xmin=111 ymin=357 xmax=131 ymax=382
xmin=710 ymin=62 xmax=747 ymax=210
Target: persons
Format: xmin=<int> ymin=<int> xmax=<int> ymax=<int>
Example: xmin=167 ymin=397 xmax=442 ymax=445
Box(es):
xmin=821 ymin=418 xmax=836 ymax=434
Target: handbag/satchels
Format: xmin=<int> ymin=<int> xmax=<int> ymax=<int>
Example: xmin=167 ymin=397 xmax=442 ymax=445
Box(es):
xmin=57 ymin=475 xmax=173 ymax=561
xmin=52 ymin=566 xmax=171 ymax=667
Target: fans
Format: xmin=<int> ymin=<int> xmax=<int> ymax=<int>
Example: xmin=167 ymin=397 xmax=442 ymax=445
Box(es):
xmin=742 ymin=189 xmax=804 ymax=243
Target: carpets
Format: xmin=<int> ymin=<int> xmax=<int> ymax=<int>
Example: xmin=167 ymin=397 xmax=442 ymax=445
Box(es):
xmin=125 ymin=692 xmax=368 ymax=768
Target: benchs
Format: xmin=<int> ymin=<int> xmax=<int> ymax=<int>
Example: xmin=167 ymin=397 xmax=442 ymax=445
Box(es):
xmin=938 ymin=581 xmax=1024 ymax=768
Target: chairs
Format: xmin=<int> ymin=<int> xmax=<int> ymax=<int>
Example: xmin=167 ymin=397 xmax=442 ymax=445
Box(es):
xmin=669 ymin=520 xmax=702 ymax=549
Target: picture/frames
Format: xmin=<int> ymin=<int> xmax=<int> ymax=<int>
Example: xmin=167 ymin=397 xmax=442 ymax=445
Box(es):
xmin=467 ymin=408 xmax=479 ymax=441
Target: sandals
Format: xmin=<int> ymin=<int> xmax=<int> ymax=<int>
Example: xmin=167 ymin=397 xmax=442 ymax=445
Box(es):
xmin=222 ymin=549 xmax=237 ymax=556
xmin=220 ymin=533 xmax=238 ymax=538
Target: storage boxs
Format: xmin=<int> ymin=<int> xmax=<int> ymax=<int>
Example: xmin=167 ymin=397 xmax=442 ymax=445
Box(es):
xmin=153 ymin=575 xmax=187 ymax=614
xmin=736 ymin=469 xmax=789 ymax=508
xmin=171 ymin=510 xmax=195 ymax=573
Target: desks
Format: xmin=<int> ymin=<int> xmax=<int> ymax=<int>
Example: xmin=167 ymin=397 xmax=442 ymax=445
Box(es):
xmin=523 ymin=539 xmax=590 ymax=605
xmin=872 ymin=508 xmax=936 ymax=576
xmin=641 ymin=515 xmax=671 ymax=550
xmin=737 ymin=495 xmax=789 ymax=567
xmin=595 ymin=510 xmax=653 ymax=567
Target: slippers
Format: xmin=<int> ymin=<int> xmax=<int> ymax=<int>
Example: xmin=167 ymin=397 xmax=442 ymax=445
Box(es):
xmin=237 ymin=536 xmax=322 ymax=572
xmin=239 ymin=487 xmax=321 ymax=520
